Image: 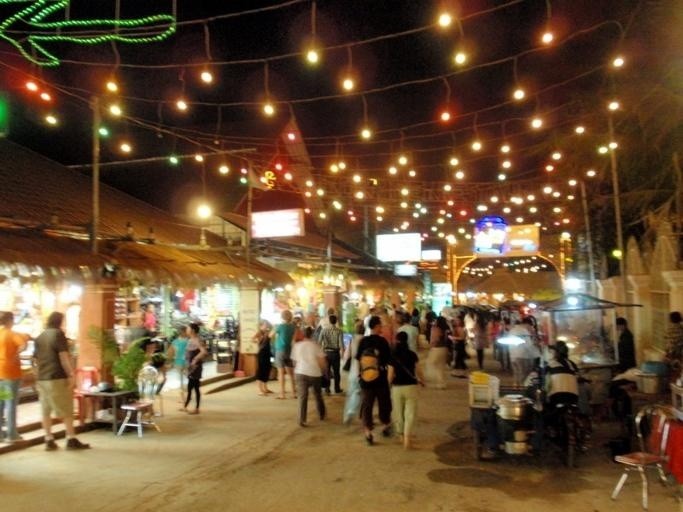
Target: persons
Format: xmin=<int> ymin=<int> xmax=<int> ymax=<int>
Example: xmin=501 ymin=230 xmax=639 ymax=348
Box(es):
xmin=425 ymin=305 xmax=548 ymax=389
xmin=248 ymin=310 xmax=304 ymax=399
xmin=0 ymin=311 xmax=28 ymax=440
xmin=33 ymin=311 xmax=89 ymax=451
xmin=539 ymin=340 xmax=595 ymax=450
xmin=666 ymin=311 xmax=683 ymax=365
xmin=138 ymin=304 xmax=169 ymax=394
xmin=169 ymin=323 xmax=209 ymax=413
xmin=288 ymin=307 xmax=346 ymax=426
xmin=616 ymin=317 xmax=637 ymax=421
xmin=349 ymin=303 xmax=424 ymax=449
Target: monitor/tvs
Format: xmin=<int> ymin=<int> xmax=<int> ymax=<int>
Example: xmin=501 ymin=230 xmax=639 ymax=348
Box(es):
xmin=252 ymin=209 xmax=305 ymax=238
xmin=376 ymin=233 xmax=422 ymax=262
xmin=422 ymin=250 xmax=441 ymax=261
xmin=393 ymin=265 xmax=418 ymax=277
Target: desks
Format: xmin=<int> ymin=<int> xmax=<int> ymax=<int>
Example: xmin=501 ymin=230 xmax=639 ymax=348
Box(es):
xmin=76 ymin=388 xmax=139 ymax=434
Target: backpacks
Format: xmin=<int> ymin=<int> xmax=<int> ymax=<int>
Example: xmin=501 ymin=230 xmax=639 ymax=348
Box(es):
xmin=359 ymin=337 xmax=384 ymax=385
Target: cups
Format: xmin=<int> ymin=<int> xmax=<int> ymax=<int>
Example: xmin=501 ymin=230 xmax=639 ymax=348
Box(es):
xmin=90 ymin=382 xmax=107 ymax=391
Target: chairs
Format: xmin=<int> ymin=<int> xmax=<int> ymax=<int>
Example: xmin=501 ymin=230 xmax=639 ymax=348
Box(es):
xmin=73 ymin=367 xmax=96 ymax=420
xmin=609 ymin=406 xmax=672 ymax=510
xmin=119 ymin=367 xmax=162 ymax=436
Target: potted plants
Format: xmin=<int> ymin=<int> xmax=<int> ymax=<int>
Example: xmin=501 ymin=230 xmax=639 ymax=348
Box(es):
xmin=90 ymin=328 xmax=150 ymax=432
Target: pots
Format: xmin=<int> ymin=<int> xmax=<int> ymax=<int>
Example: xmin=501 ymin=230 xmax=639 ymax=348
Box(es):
xmin=514 ymin=429 xmax=537 ymax=442
xmin=495 ymin=394 xmax=536 ymax=419
xmin=504 ymin=439 xmax=530 ymax=456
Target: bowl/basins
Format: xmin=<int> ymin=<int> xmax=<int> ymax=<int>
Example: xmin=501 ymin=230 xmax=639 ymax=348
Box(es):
xmin=633 ymin=375 xmax=667 ymax=395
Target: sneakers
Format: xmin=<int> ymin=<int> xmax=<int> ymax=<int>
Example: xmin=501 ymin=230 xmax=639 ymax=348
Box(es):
xmin=4 ymin=435 xmax=24 ymax=445
xmin=178 ymin=406 xmax=201 ymax=416
xmin=256 ymin=384 xmax=347 ymax=426
xmin=45 ymin=439 xmax=59 ymax=452
xmin=363 ymin=424 xmax=415 ymax=450
xmin=66 ymin=438 xmax=90 ymax=451
